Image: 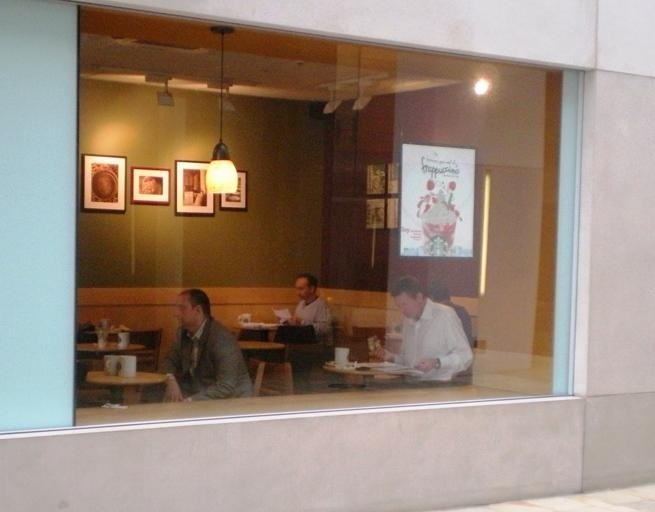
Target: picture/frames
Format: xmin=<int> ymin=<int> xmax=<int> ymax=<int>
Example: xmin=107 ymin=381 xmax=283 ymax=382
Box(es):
xmin=81 ymin=153 xmax=248 ymax=218
xmin=363 ymin=160 xmax=401 ymax=230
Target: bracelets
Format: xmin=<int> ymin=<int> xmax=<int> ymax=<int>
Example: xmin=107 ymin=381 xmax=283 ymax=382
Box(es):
xmin=435 ymin=358 xmax=441 ymax=369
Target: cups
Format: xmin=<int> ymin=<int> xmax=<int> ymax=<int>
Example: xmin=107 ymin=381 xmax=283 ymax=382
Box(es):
xmin=335 ymin=348 xmax=351 ymax=365
xmin=104 ymin=355 xmax=137 ymax=379
xmin=238 ymin=314 xmax=251 ymax=325
xmin=117 ymin=333 xmax=130 ymax=348
xmin=422 ymin=217 xmax=457 ymax=248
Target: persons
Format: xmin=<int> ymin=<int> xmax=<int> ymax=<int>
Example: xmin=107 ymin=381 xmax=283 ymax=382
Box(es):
xmin=426 ymin=278 xmax=473 ymax=385
xmin=269 ymin=272 xmax=334 ymax=394
xmin=157 ymin=289 xmax=254 ymax=404
xmin=363 ymin=274 xmax=474 ymax=392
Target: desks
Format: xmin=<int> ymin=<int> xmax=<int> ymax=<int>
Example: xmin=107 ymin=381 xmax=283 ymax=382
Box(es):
xmin=231 ymin=320 xmax=287 ymax=358
xmin=322 ymin=359 xmax=404 ymax=390
xmin=76 ymin=341 xmax=167 ymax=406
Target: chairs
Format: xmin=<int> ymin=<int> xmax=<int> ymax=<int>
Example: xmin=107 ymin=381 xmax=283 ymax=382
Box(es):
xmin=120 ymin=327 xmax=163 ymax=373
xmin=244 ymin=358 xmax=295 ymax=397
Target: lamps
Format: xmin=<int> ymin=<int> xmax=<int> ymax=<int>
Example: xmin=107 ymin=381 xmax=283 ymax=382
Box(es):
xmin=205 ymin=24 xmax=239 ymax=196
